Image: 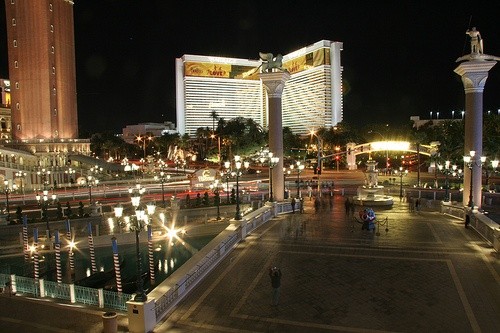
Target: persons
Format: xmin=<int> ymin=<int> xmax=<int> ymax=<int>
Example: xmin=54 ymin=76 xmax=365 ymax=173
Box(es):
xmin=465 ymin=201 xmax=474 ymax=228
xmin=300 ymin=198 xmax=305 ymax=214
xmin=315 ymin=197 xmax=333 ymax=214
xmin=415 ymin=199 xmax=419 ymax=211
xmin=344 ymin=198 xmax=351 ymax=220
xmin=291 ymin=198 xmax=295 ymax=214
xmin=269 ymin=264 xmax=281 ymax=306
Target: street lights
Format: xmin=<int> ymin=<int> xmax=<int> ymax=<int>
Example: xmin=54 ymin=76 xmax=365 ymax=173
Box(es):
xmin=438 ymin=160 xmax=458 ymax=202
xmin=259 ymin=149 xmax=280 ymax=202
xmin=36 ymin=168 xmax=50 ymax=190
xmin=153 ymin=171 xmax=171 ymax=209
xmin=224 ymin=154 xmax=250 ymax=220
xmin=80 ymin=175 xmax=99 ymax=206
xmin=290 ymin=161 xmax=306 ymax=200
xmin=14 ymin=170 xmax=27 ymax=195
xmin=35 ymin=189 xmax=57 ymax=230
xmin=394 ymin=167 xmax=408 ymax=198
xmin=114 ymin=191 xmax=157 ymax=302
xmin=282 ymin=166 xmax=291 ymax=199
xmin=462 ymin=149 xmax=486 ymax=208
xmin=3 ymin=178 xmax=17 ymax=221
